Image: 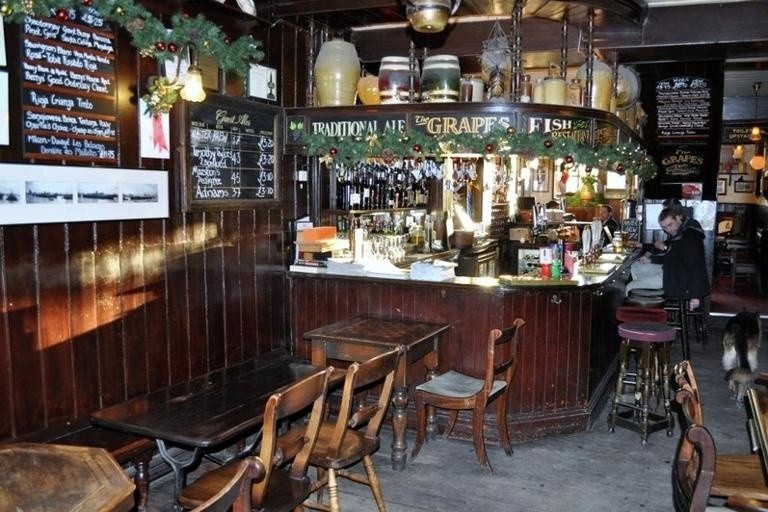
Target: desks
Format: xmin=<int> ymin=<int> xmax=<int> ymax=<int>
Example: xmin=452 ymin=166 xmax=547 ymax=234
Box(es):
xmin=301 ymin=311 xmax=452 ymax=472
xmin=398 ymin=248 xmax=461 ymax=267
xmin=90 ymin=346 xmax=349 ymax=511
xmin=0 ymin=440 xmax=139 ymax=511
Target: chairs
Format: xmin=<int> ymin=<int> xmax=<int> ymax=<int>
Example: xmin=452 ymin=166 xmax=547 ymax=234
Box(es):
xmin=176 ymin=365 xmax=336 ymax=512
xmin=191 ymin=454 xmax=264 ymax=512
xmin=408 ymin=315 xmax=526 ymax=479
xmin=716 ymin=227 xmax=765 ymax=295
xmin=277 ymin=343 xmax=407 ymax=512
xmin=670 ymin=358 xmax=768 ymax=512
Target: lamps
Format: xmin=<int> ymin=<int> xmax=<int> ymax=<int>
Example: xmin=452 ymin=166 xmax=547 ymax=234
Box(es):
xmin=748 ymin=126 xmax=768 ymax=142
xmin=404 ymin=0 xmax=453 ymax=36
xmin=732 ymin=146 xmax=744 ymax=163
xmin=141 ymin=40 xmax=207 ymax=116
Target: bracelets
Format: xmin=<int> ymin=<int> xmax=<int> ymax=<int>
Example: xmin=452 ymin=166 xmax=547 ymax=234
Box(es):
xmin=664 ymin=245 xmax=667 ymax=251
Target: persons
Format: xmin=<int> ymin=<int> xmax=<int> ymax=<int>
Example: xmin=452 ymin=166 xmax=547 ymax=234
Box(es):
xmin=546 ymin=200 xmax=560 ymax=209
xmin=624 ymin=208 xmax=710 ymax=313
xmin=642 ymin=197 xmax=703 ymax=257
xmin=600 ymin=205 xmax=618 ymax=247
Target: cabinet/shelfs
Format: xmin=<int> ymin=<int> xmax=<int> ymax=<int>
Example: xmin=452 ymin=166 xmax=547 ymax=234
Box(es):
xmin=429 ymin=159 xmax=452 ymax=213
xmin=471 ymin=158 xmax=491 ymax=223
xmin=320 ymin=158 xmax=428 ymax=213
xmin=462 ymin=240 xmax=500 ymax=277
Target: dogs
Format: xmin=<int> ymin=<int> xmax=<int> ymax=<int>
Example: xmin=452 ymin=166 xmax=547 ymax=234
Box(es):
xmin=721 ymin=305 xmax=763 ymax=410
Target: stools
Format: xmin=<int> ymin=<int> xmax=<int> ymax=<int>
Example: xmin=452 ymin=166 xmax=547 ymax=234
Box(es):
xmin=606 ymin=291 xmax=710 ymax=448
xmin=43 ymin=427 xmax=158 ymax=512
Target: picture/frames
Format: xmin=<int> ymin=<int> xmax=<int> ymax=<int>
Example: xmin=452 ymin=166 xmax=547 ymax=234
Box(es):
xmin=734 ymin=180 xmax=754 ymax=194
xmin=717 ymin=177 xmax=727 ymax=196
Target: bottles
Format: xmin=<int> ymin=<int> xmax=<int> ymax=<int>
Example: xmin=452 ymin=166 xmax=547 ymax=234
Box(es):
xmin=544 ymin=61 xmax=568 ymax=106
xmin=472 ymin=75 xmax=485 ymax=102
xmin=335 ymin=165 xmax=429 ymax=211
xmin=520 ymin=73 xmax=532 ymax=103
xmin=567 ymin=78 xmax=584 ymax=106
xmin=552 ymin=243 xmax=562 ymax=279
xmin=533 ymin=77 xmax=545 ymax=103
xmin=460 ymin=76 xmax=473 ymax=103
xmin=411 ymin=225 xmax=425 ymax=246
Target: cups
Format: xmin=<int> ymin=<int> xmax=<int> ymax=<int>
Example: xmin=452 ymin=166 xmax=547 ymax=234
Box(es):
xmin=683 ymin=185 xmax=700 ymax=200
xmin=613 ymin=238 xmax=623 ymax=253
xmin=614 ymin=231 xmax=621 ymax=238
xmin=621 ymin=231 xmax=629 ymax=247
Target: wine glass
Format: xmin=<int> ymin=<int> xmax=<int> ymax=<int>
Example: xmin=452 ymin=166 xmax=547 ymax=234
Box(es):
xmin=368 ymin=232 xmax=408 ymax=261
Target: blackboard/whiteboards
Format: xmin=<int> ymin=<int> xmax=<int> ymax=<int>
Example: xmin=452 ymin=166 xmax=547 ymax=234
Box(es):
xmin=172 ymin=90 xmax=285 ymax=213
